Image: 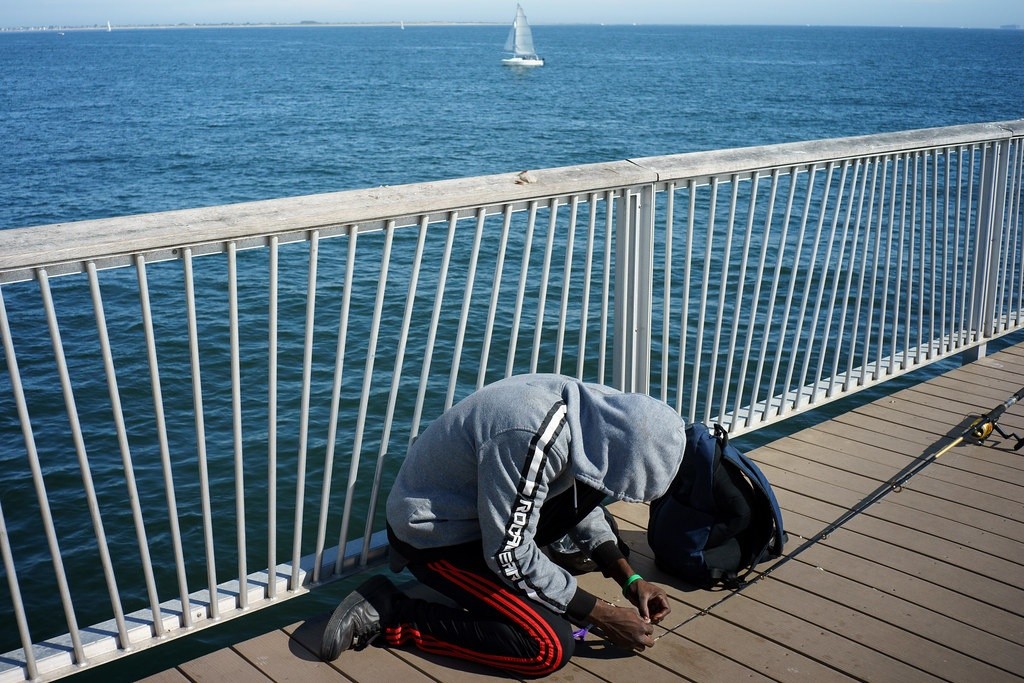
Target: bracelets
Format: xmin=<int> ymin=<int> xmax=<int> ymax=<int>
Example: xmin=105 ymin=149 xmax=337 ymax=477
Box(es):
xmin=622 ymin=574 xmax=643 ymax=599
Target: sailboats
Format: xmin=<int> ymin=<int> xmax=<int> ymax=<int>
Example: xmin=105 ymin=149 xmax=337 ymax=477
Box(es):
xmin=500 ymin=3 xmax=546 ymax=67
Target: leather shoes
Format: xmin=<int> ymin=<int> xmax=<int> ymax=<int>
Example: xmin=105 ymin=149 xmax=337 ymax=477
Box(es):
xmin=321 ymin=574 xmax=406 ymax=662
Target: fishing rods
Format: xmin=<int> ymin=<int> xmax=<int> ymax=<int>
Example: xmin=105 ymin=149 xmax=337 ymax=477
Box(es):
xmin=645 ymin=353 xmax=1023 ymax=645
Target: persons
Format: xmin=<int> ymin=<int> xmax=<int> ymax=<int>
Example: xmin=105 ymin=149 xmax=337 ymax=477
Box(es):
xmin=319 ymin=374 xmax=687 ymax=679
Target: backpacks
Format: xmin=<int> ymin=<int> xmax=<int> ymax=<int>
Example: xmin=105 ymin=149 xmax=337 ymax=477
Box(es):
xmin=647 ymin=423 xmax=789 ymax=590
